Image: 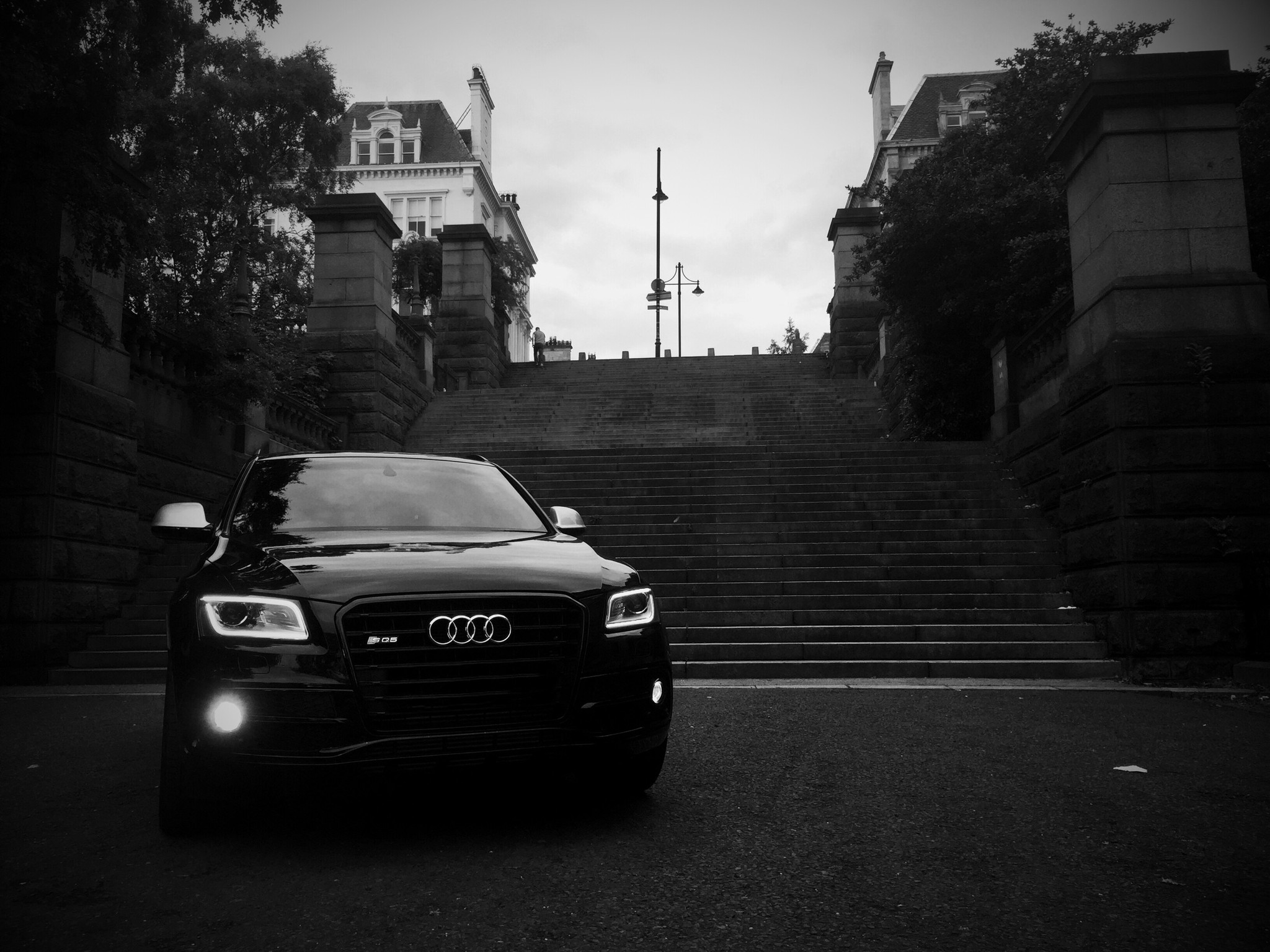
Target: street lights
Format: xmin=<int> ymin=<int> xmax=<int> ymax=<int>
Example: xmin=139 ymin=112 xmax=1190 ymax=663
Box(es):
xmin=663 ymin=262 xmax=705 ymax=357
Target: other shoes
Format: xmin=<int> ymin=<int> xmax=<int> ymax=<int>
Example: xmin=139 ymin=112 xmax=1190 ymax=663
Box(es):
xmin=540 ymin=364 xmax=543 ymax=366
xmin=535 ymin=363 xmax=538 ymax=365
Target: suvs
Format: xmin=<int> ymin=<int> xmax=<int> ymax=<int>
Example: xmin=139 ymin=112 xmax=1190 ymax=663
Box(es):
xmin=150 ymin=449 xmax=675 ymax=836
